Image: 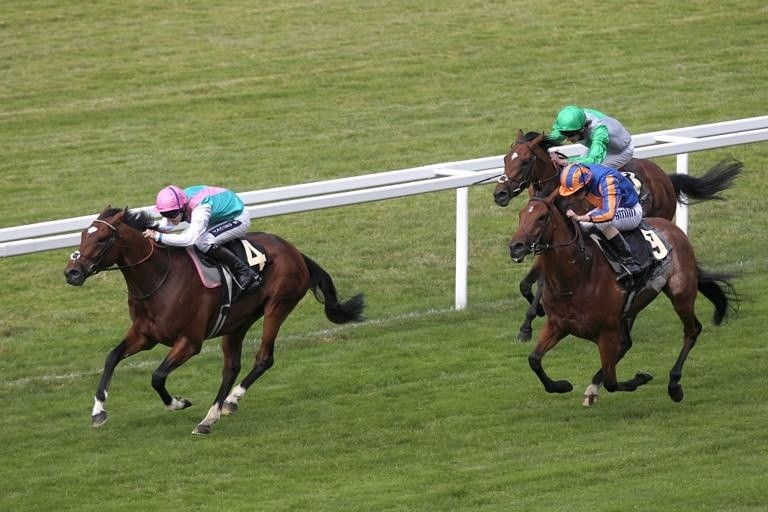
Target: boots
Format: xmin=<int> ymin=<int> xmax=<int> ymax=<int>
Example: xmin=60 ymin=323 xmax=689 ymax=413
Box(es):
xmin=608 ymin=232 xmax=641 ymax=281
xmin=216 ymin=246 xmax=256 ymax=296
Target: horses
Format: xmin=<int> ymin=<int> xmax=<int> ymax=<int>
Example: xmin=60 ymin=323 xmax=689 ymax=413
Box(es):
xmin=492 ymin=128 xmax=746 ymax=342
xmin=63 ymin=203 xmax=369 ymax=437
xmin=508 ymin=182 xmax=746 ymax=408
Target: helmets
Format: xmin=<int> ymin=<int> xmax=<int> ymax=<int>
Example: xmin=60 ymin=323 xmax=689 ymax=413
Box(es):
xmin=156 ymin=185 xmax=188 ymax=212
xmin=552 ymin=106 xmax=586 ymax=131
xmin=559 ymin=162 xmax=592 ymax=196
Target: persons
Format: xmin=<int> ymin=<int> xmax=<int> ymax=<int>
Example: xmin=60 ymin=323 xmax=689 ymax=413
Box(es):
xmin=546 ymin=104 xmax=636 ymax=171
xmin=143 ymin=182 xmax=262 ymax=300
xmin=560 ymin=160 xmax=644 ymax=285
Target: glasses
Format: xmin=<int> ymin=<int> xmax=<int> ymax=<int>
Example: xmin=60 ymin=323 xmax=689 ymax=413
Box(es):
xmin=560 ymin=131 xmax=578 ymax=137
xmin=573 ymin=188 xmax=584 ymax=197
xmin=160 ymin=210 xmax=182 ymax=218
